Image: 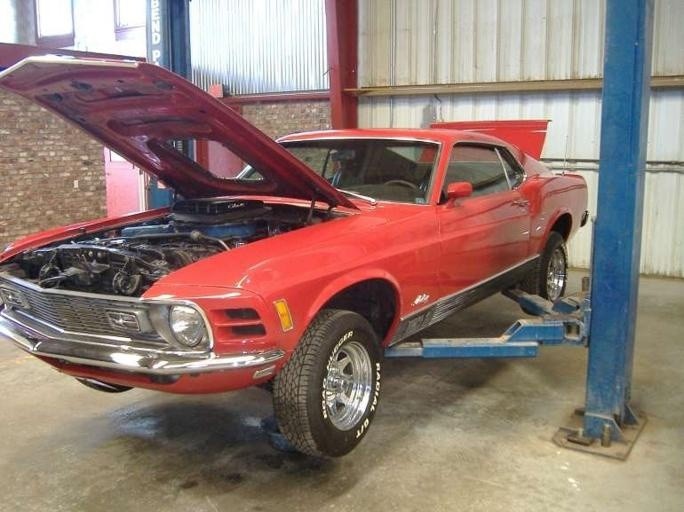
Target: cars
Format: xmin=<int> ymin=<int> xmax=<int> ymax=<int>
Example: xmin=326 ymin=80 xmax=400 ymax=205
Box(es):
xmin=0 ymin=39 xmax=591 ymax=463
